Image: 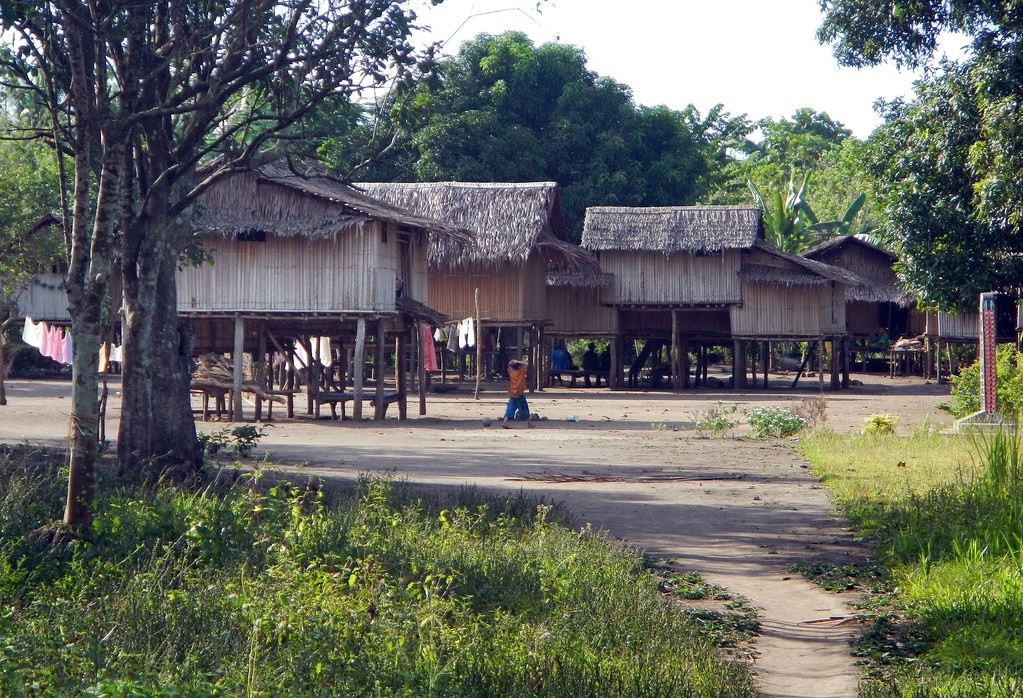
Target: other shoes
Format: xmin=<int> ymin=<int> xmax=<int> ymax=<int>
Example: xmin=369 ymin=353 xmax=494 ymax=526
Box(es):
xmin=528 ymin=423 xmax=535 ymax=428
xmin=501 ymin=423 xmax=514 ymax=429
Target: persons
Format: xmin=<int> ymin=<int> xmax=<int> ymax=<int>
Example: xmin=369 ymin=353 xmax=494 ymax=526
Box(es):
xmin=869 ymin=325 xmax=889 ymax=351
xmin=500 ymin=359 xmax=538 ymax=429
xmin=582 ymin=342 xmax=602 ymax=387
xmin=549 ymin=342 xmax=577 ymax=387
xmin=599 ymin=344 xmax=613 ymax=387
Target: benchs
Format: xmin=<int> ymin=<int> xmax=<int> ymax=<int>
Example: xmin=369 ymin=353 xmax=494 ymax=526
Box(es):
xmin=548 ymin=368 xmax=611 ymax=386
